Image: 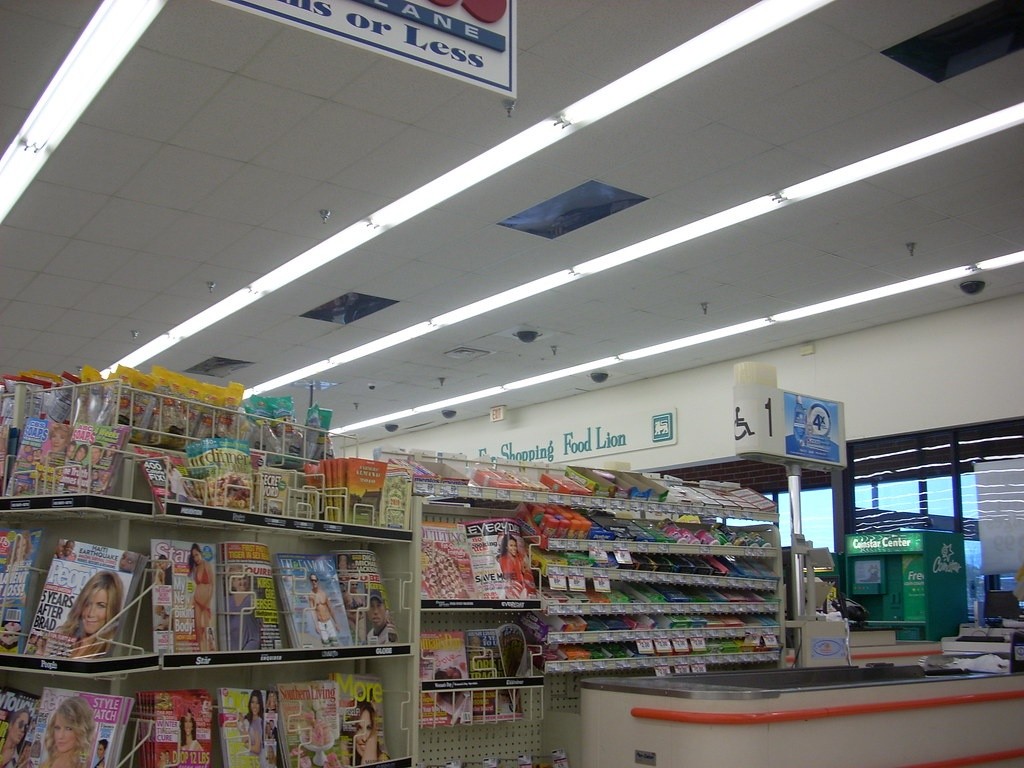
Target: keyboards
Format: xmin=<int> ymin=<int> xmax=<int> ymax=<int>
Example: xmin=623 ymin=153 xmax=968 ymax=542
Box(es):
xmin=956 ymin=636 xmax=1005 ymax=643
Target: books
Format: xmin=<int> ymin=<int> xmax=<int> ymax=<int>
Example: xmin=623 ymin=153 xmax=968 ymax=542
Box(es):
xmin=420 ymin=518 xmax=536 ymax=725
xmin=0 ymin=417 xmax=398 ymax=768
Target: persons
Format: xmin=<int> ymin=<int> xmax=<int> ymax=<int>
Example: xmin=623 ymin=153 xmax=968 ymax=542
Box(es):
xmin=496 ymin=533 xmax=534 ymax=599
xmin=364 ymin=591 xmax=398 ymax=644
xmin=239 ymin=690 xmax=266 ymax=767
xmin=188 ymin=544 xmax=214 ymax=652
xmin=347 ymin=699 xmax=390 ymax=766
xmin=58 ymin=570 xmax=123 ymax=659
xmin=228 ymin=565 xmax=261 ymax=650
xmin=309 ymin=574 xmax=341 ymax=647
xmin=39 ymin=697 xmax=95 ymax=768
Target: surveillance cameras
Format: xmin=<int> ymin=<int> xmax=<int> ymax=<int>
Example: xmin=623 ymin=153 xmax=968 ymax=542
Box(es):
xmin=442 ymin=410 xmax=456 ymax=419
xmin=591 ymin=373 xmax=608 ymax=383
xmin=960 ymin=281 xmax=985 ymax=294
xmin=517 ymin=331 xmax=537 ymax=343
xmin=385 ymin=424 xmax=398 ymax=432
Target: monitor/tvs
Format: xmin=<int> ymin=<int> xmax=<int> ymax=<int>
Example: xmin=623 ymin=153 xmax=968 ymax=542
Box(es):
xmin=983 ymin=589 xmax=1019 ymax=620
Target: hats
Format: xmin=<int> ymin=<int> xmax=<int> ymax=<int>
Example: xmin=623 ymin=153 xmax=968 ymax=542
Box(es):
xmin=370 ymin=589 xmax=383 ymax=604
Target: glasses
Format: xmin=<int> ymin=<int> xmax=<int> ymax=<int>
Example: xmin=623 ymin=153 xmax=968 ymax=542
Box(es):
xmin=311 ymin=579 xmax=318 ymax=582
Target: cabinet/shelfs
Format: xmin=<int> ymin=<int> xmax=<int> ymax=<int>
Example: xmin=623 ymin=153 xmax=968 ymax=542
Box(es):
xmin=0 ymin=365 xmax=786 ymax=768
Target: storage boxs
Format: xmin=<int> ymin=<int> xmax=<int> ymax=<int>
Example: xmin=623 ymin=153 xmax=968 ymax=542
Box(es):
xmin=701 ymin=557 xmax=728 ymax=576
xmin=418 ymin=461 xmax=470 ymax=485
xmin=631 ymin=555 xmax=656 ymax=572
xmin=589 ymin=468 xmax=632 ymax=500
xmin=665 ymin=554 xmax=695 ymax=574
xmin=685 ymin=557 xmax=714 ymax=575
xmin=526 ymin=545 xmax=569 ymax=575
xmin=541 ymin=474 xmax=588 ymax=496
xmin=389 ymin=458 xmax=441 ymax=483
xmin=565 ymin=466 xmax=616 ymax=498
xmin=645 ymin=555 xmax=674 ymax=573
xmin=607 ymin=470 xmax=652 ymax=502
xmin=624 ymin=472 xmax=669 ymax=503
